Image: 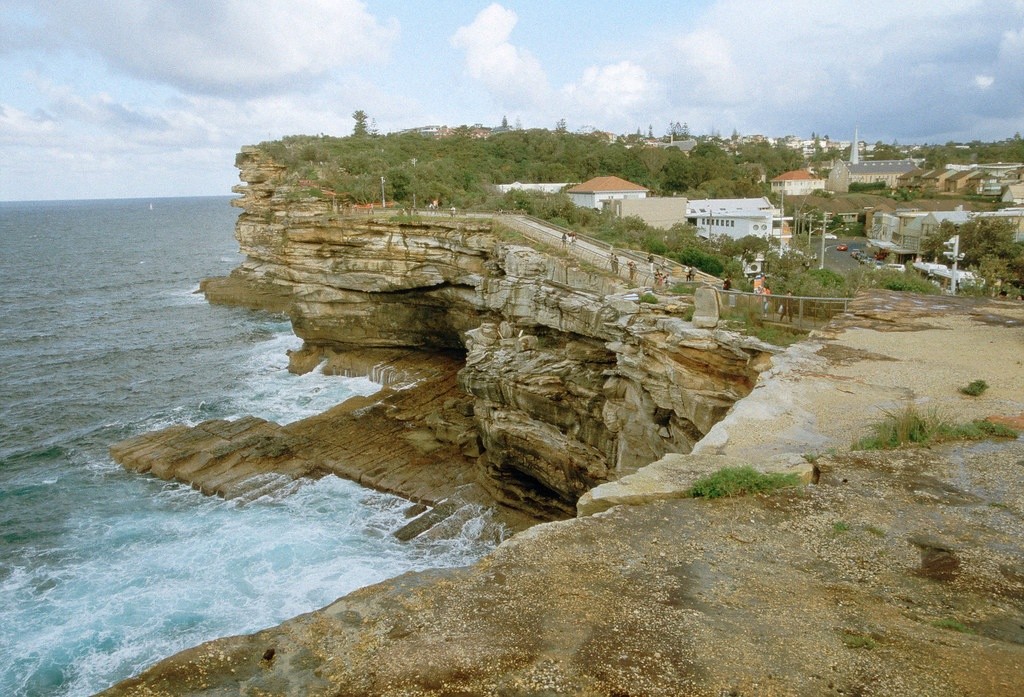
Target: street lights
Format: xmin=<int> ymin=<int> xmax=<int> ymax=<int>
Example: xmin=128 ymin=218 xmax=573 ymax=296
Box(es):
xmin=798 ymin=209 xmax=819 ymax=235
xmin=808 ymin=226 xmax=830 ymax=249
xmin=942 ymin=235 xmax=965 ymax=293
xmin=793 ymin=188 xmax=834 ymax=253
xmin=821 ymin=228 xmax=850 ymax=268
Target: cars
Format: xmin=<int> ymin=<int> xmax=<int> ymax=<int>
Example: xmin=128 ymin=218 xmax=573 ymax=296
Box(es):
xmin=851 ymin=249 xmax=882 ymax=269
xmin=825 ymin=233 xmax=837 ymax=240
xmin=837 ymin=244 xmax=848 ymax=251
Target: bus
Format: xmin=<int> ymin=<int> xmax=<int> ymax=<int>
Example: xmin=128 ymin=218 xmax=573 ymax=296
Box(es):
xmin=913 ymin=262 xmax=985 ymax=295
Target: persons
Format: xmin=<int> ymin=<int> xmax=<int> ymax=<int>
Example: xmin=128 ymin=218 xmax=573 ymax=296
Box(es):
xmin=336 ymin=197 xmax=374 ymax=214
xmin=429 ymin=200 xmax=456 ymax=217
xmin=647 ymin=252 xmax=671 ymax=287
xmin=723 ymin=275 xmax=730 ymax=290
xmin=761 ymin=286 xmax=793 ymax=322
xmin=561 ymin=232 xmax=575 ymax=246
xmin=610 ymin=253 xmax=637 ymax=281
xmin=685 ymin=264 xmax=696 ymax=281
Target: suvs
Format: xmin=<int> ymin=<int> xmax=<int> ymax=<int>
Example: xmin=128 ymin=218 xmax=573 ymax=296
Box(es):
xmin=877 ymin=263 xmax=905 ymax=274
